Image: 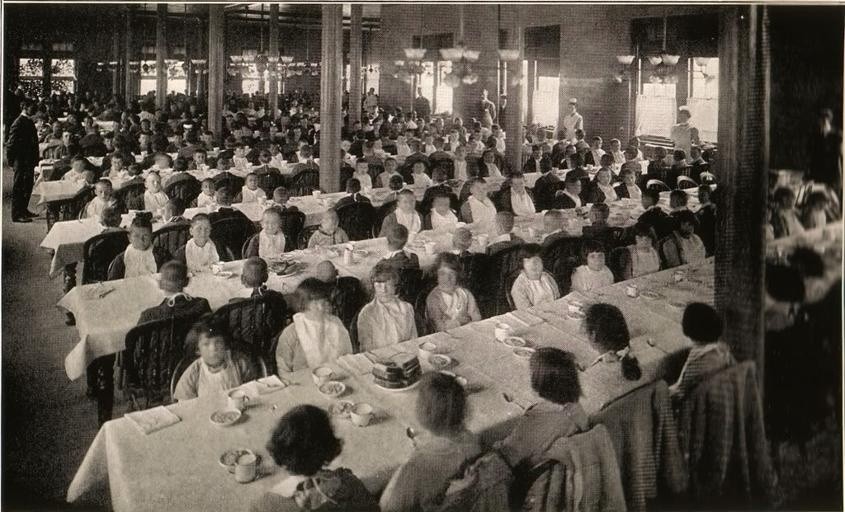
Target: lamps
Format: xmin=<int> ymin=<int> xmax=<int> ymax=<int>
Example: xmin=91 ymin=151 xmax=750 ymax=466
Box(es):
xmin=695 ymin=57 xmax=715 ymax=84
xmin=439 ymin=48 xmax=464 ymax=89
xmin=96 ymin=3 xmax=322 ymax=77
xmin=613 ymin=55 xmax=635 ymax=83
xmin=404 ymin=48 xmax=426 ymax=73
xmin=394 ymin=61 xmax=405 ymax=70
xmin=462 ymin=50 xmax=480 ymax=85
xmin=496 ymin=49 xmax=521 ymax=88
xmin=648 ymin=7 xmax=680 ymax=66
xmin=360 ymin=26 xmax=380 ymax=80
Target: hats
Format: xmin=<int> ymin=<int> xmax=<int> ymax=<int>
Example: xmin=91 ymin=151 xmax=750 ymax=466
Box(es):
xmin=569 ymin=97 xmax=577 ymax=103
xmin=679 ymin=105 xmax=690 ymax=111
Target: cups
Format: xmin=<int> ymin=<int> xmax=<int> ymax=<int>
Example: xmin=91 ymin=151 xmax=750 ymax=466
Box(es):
xmin=350 ymin=403 xmax=375 ymax=427
xmin=793 ymin=225 xmax=835 ymax=256
xmin=568 ymin=300 xmax=583 ymax=311
xmin=623 ymin=284 xmax=638 ymax=297
xmin=210 ymin=231 xmax=436 ymax=276
xmin=675 ymin=270 xmax=684 ymax=283
xmin=312 ymin=367 xmax=336 ymax=386
xmin=234 ymin=454 xmax=257 ymax=483
xmin=418 ymin=342 xmax=436 ymax=359
xmin=227 ymin=389 xmax=250 ymax=412
xmin=495 ymin=322 xmax=512 ymax=342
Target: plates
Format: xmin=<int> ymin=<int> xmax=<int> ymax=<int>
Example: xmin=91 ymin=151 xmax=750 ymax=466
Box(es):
xmin=318 ymin=381 xmax=346 ymax=397
xmin=668 ymin=301 xmax=687 ymax=310
xmin=376 ymin=379 xmax=421 ymax=392
xmin=328 ymin=400 xmax=354 ymax=417
xmin=503 ymin=337 xmax=526 ymax=347
xmin=426 ymin=354 xmax=452 ymax=368
xmin=567 ymin=310 xmax=586 ymax=320
xmin=210 ymin=408 xmax=241 ymax=425
xmin=513 ymin=347 xmax=536 ymax=359
xmin=219 ymin=448 xmax=253 ymax=474
xmin=639 ymin=291 xmax=659 ymax=300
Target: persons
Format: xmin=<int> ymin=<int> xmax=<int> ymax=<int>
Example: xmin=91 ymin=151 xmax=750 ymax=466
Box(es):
xmin=357 ymin=262 xmax=418 ymax=352
xmin=276 ymin=278 xmax=354 ymax=376
xmin=575 ymin=304 xmax=651 ymax=418
xmin=491 ymin=345 xmax=591 ymax=472
xmin=173 ymin=314 xmax=260 ymax=403
xmin=668 ymin=302 xmax=739 ymax=399
xmin=2 ymin=83 xmax=843 ymax=332
xmin=248 ymin=404 xmax=381 ymax=510
xmin=379 ymin=370 xmax=482 ymax=512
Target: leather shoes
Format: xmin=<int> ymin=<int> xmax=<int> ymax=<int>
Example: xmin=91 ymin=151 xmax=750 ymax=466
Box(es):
xmin=14 ymin=210 xmax=39 ymax=223
